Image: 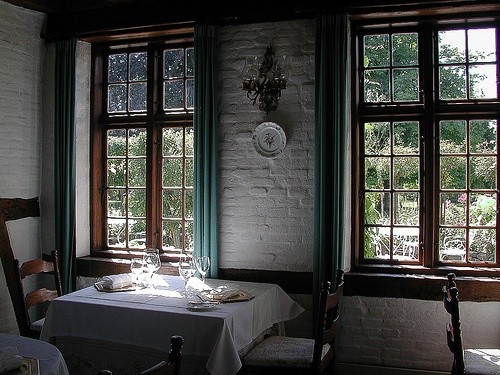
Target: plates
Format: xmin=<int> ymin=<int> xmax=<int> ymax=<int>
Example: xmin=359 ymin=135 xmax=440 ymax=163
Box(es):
xmin=94 ymin=281 xmax=136 ymax=292
xmin=197 ymin=289 xmax=256 ymax=303
xmin=251 ymin=121 xmax=287 ymax=159
xmin=16 ymin=355 xmax=41 ymax=375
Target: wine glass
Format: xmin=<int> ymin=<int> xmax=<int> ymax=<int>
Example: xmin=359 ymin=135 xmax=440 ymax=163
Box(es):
xmin=143 ymin=253 xmax=162 ymax=288
xmin=130 ymin=258 xmax=143 ymax=290
xmin=179 ymin=257 xmax=192 ymax=299
xmin=195 ymin=256 xmax=210 ymax=286
xmin=144 ymin=249 xmax=159 ymax=290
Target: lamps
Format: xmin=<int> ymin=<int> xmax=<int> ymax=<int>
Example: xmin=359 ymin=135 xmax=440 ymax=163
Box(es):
xmin=239 ymin=47 xmax=292 ymax=118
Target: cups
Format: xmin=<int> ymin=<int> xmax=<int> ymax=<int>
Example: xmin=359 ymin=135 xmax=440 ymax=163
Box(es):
xmin=190 ymin=259 xmax=197 ymax=277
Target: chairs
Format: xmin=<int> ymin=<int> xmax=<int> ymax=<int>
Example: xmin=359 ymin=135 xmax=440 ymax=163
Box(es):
xmin=99 ymin=333 xmax=185 ymax=375
xmin=13 ymin=249 xmax=63 ymax=344
xmin=442 ymin=272 xmax=500 ymax=375
xmin=0 ymin=332 xmax=69 ymax=375
xmin=241 ymin=269 xmax=347 ymax=375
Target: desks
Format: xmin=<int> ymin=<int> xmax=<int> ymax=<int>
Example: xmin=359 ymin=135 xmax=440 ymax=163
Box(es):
xmin=37 ymin=272 xmax=307 ymax=375
